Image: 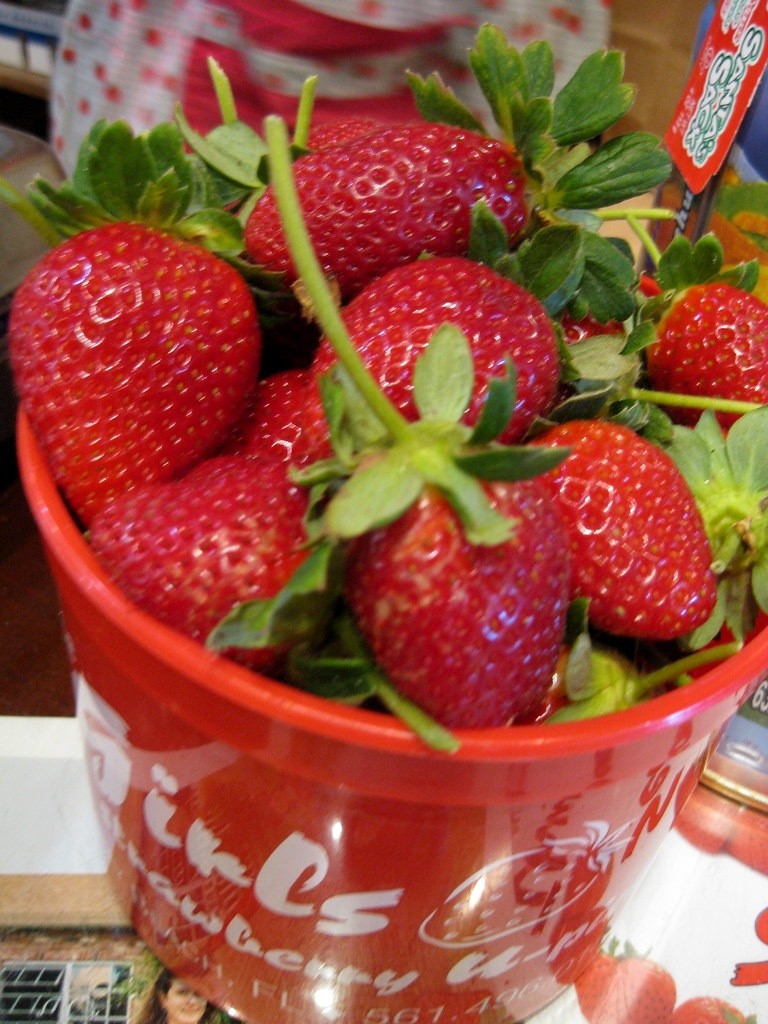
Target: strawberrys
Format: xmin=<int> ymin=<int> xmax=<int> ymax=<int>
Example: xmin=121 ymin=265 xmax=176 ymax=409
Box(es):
xmin=2 ymin=26 xmax=768 ymax=749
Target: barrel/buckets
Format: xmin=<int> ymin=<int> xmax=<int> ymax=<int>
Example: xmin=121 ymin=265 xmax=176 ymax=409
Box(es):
xmin=17 ymin=227 xmax=768 ymax=1023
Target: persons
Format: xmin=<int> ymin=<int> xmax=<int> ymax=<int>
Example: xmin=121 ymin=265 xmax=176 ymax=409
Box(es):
xmin=134 ymin=962 xmax=216 ymax=1024
